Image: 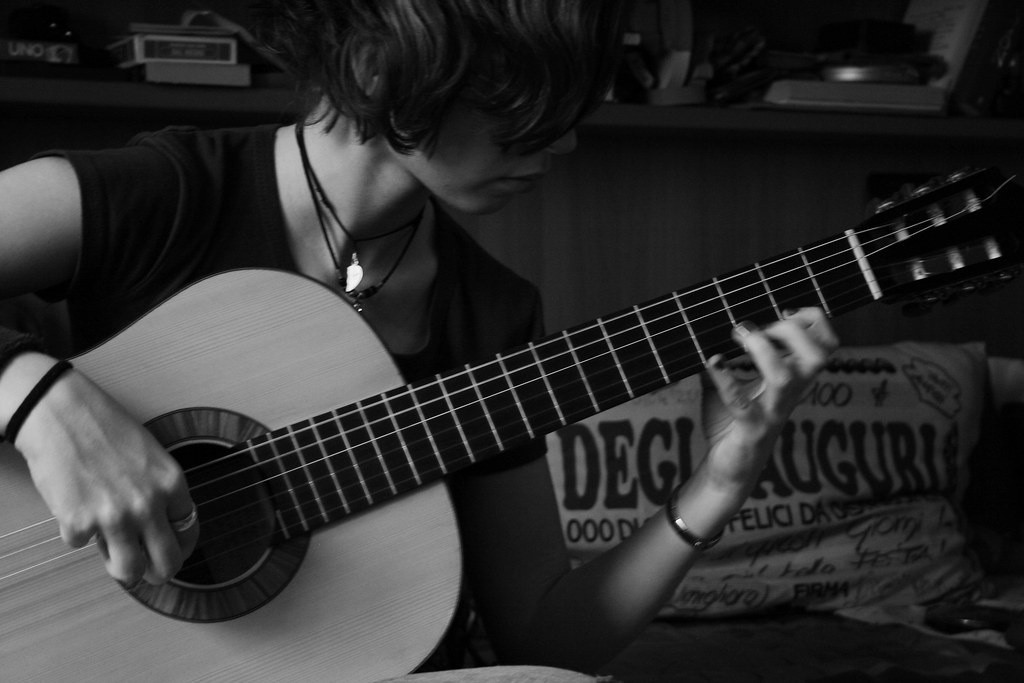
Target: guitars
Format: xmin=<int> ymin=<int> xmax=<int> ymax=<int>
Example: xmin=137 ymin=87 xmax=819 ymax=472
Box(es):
xmin=0 ymin=158 xmax=1023 ymax=683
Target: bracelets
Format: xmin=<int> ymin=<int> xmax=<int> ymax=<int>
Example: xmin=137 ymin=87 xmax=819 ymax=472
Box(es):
xmin=665 ymin=483 xmax=725 ymax=551
xmin=4 ymin=361 xmax=75 ymax=443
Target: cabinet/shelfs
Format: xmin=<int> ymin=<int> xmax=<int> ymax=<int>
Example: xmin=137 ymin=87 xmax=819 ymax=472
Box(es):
xmin=0 ymin=74 xmax=1023 ymax=147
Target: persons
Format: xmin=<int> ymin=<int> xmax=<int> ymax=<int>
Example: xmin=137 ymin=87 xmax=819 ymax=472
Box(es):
xmin=0 ymin=0 xmax=838 ymax=683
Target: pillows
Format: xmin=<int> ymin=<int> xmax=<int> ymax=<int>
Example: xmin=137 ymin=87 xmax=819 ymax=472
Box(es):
xmin=545 ymin=339 xmax=998 ymax=621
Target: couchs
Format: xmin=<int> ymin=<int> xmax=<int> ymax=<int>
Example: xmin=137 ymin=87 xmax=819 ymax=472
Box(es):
xmin=464 ymin=359 xmax=1021 ymax=682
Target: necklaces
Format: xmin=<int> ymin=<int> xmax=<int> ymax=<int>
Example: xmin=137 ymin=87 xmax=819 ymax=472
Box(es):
xmin=294 ymin=116 xmax=428 ymax=314
